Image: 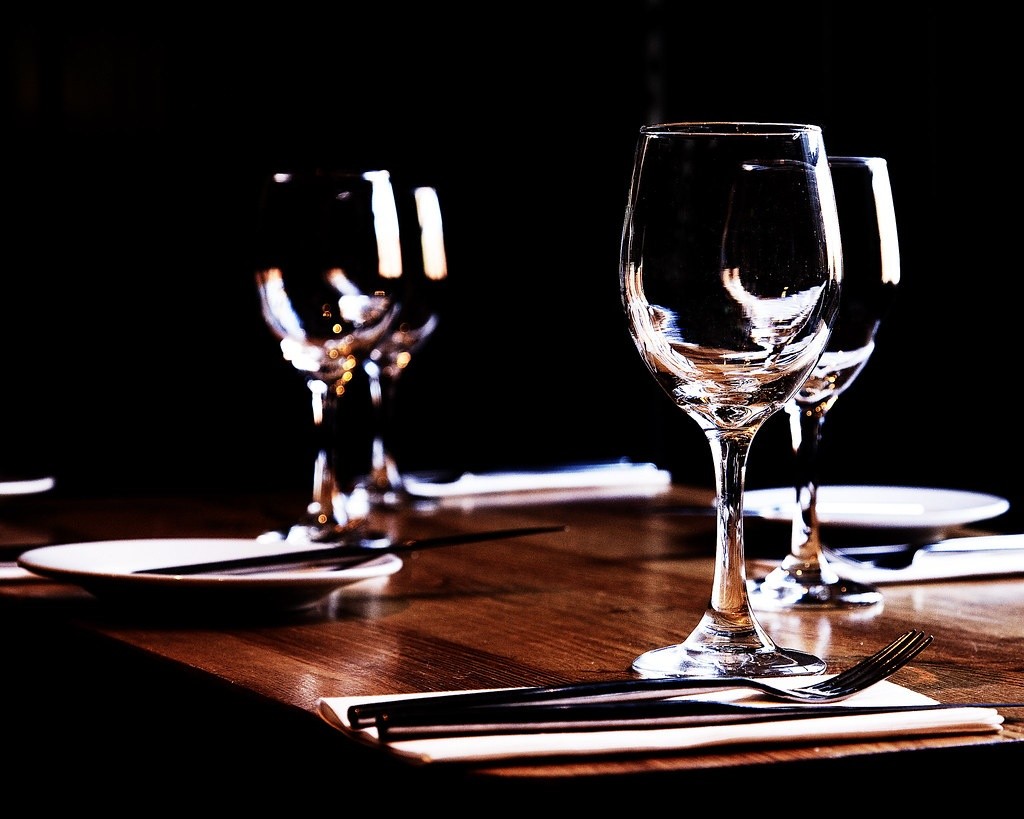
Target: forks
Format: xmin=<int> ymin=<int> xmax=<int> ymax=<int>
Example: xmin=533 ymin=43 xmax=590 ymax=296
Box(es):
xmin=816 ymin=534 xmax=932 ymax=571
xmin=346 ymin=625 xmax=935 ymax=729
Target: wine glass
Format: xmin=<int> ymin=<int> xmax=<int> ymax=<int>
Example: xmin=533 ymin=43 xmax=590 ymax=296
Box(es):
xmin=615 ymin=115 xmax=846 ymax=679
xmin=717 ymin=149 xmax=902 ymax=611
xmin=252 ymin=162 xmax=401 ymax=551
xmin=322 ymin=183 xmax=450 ymax=516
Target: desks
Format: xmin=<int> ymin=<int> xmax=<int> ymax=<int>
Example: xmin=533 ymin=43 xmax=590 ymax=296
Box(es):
xmin=1 ymin=487 xmax=1024 ymax=819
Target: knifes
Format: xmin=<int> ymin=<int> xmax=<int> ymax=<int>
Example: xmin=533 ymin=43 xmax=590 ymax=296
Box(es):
xmin=131 ymin=520 xmax=577 ymax=582
xmin=371 ymin=698 xmax=1024 ymax=741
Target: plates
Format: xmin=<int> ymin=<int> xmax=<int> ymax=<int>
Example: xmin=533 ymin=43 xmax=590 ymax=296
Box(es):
xmin=15 ymin=538 xmax=404 ymax=616
xmin=711 ymin=484 xmax=1009 ymax=533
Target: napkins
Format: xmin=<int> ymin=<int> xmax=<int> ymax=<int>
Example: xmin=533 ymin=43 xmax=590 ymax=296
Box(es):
xmin=406 ymin=464 xmax=670 ymax=509
xmin=317 ymin=672 xmax=1006 ymax=763
